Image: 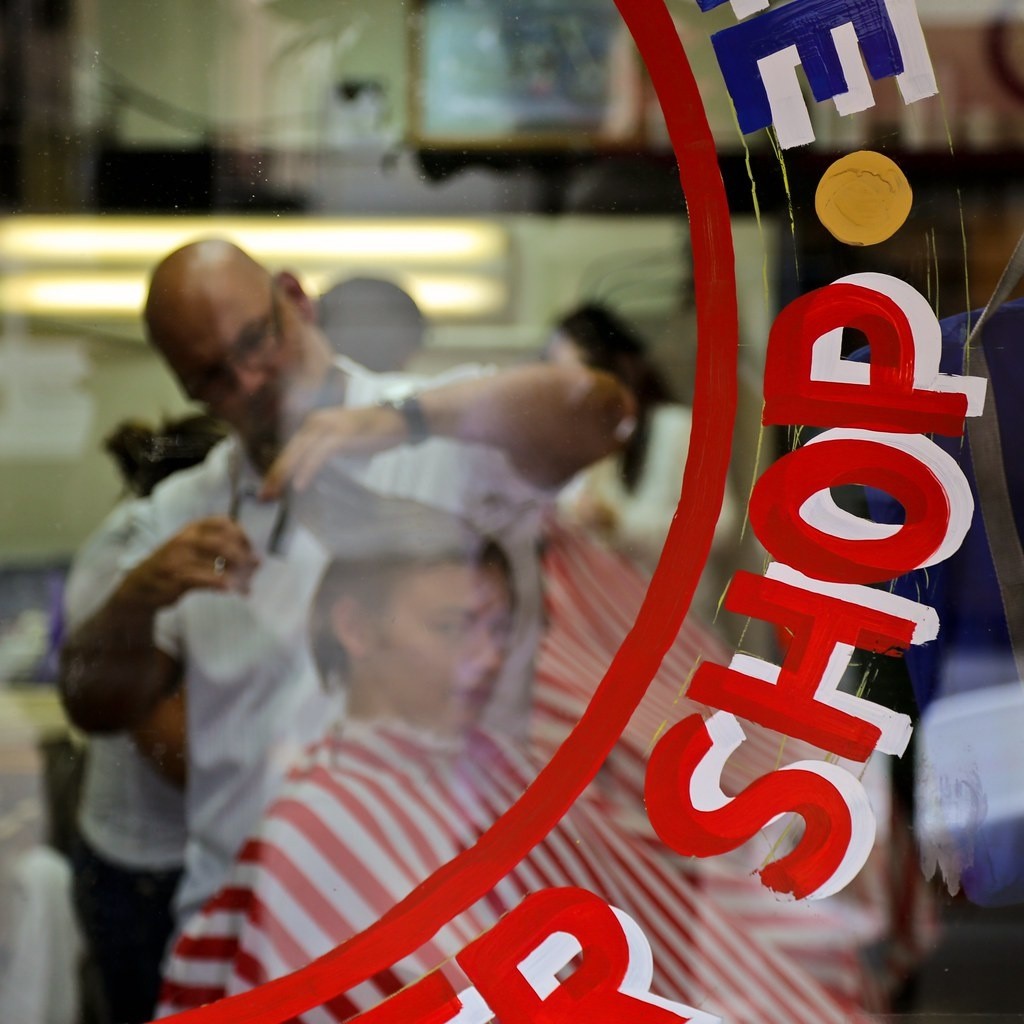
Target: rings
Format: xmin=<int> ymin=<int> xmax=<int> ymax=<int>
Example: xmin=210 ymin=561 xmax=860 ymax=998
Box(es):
xmin=210 ymin=555 xmax=225 ymax=576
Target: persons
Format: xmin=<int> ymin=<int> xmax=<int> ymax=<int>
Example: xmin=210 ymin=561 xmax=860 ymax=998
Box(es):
xmin=56 ymin=238 xmax=922 ymax=1024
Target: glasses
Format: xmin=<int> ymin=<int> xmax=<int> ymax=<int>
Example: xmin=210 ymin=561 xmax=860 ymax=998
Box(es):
xmin=186 ymin=287 xmax=281 ymax=403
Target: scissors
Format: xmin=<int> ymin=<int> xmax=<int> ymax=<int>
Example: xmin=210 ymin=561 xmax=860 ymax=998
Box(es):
xmin=214 ymin=482 xmax=243 ymax=577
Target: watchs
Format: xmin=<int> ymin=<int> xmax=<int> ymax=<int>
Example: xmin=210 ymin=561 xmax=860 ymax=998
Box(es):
xmin=374 ymin=381 xmax=431 ymax=449
